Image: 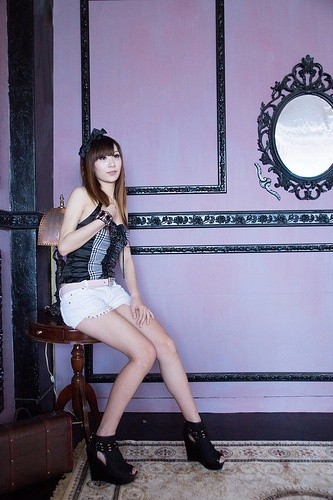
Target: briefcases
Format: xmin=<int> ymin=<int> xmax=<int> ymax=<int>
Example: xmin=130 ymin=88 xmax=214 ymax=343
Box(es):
xmin=0 ymin=411 xmax=73 ymax=497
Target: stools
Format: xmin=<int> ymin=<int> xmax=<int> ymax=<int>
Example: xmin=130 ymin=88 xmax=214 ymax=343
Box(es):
xmin=29 ymin=321 xmax=101 ymax=445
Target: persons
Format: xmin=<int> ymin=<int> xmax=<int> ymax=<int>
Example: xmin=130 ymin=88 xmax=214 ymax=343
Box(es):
xmin=57 ymin=127 xmax=226 ymax=485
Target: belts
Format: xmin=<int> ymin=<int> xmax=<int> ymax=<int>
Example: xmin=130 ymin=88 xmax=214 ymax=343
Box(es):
xmin=57 ymin=277 xmax=114 ymax=297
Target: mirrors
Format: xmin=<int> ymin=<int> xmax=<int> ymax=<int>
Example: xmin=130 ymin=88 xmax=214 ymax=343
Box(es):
xmin=257 ymin=55 xmax=333 ymax=201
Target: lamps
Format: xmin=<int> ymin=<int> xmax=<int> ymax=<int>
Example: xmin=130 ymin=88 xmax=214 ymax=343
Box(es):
xmin=38 ymin=194 xmax=66 ymax=321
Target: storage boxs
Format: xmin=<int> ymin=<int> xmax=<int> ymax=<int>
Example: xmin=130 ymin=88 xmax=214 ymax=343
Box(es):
xmin=0 ymin=408 xmax=73 ymax=497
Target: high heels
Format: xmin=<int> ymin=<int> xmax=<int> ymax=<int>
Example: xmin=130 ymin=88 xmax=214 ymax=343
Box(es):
xmin=85 ymin=436 xmax=138 ymax=484
xmin=183 ymin=419 xmax=227 ymax=471
